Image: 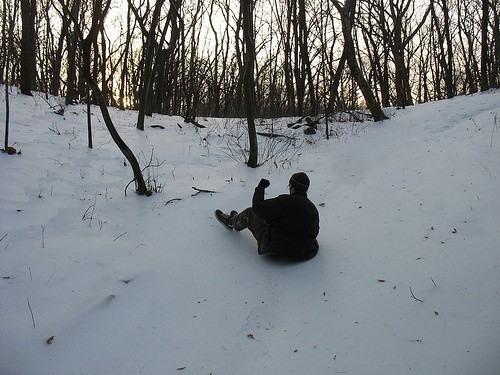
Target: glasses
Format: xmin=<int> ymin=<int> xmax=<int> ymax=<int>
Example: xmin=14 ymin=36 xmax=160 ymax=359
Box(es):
xmin=287 ymin=184 xmax=291 ymax=189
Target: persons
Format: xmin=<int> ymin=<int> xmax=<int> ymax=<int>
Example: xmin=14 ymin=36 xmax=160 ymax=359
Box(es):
xmin=214 ymin=172 xmax=320 ymax=263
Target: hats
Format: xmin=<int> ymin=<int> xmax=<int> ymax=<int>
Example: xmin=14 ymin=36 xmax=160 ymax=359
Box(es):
xmin=289 ymin=172 xmax=310 ymax=191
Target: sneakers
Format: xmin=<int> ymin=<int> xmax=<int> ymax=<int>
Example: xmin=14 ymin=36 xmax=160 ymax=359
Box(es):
xmin=215 ymin=210 xmax=233 ymax=231
xmin=230 ymin=211 xmax=241 ymax=231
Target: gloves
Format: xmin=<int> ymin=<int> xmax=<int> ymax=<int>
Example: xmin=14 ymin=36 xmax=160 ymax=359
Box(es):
xmin=257 ymin=178 xmax=270 ymax=189
